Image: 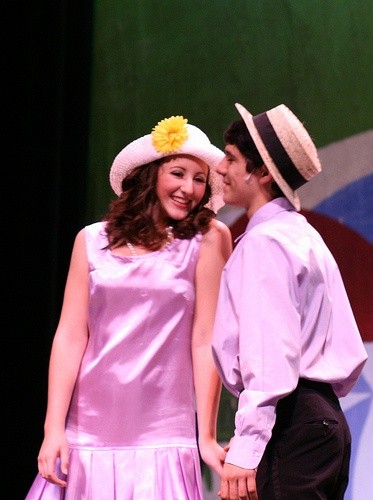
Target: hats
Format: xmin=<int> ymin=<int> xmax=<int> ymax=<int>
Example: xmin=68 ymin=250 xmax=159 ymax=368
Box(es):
xmin=110 ymin=116 xmax=226 ymax=214
xmin=234 ymin=103 xmax=321 ymax=211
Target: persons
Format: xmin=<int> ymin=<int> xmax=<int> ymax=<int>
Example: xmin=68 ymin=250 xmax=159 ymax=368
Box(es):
xmin=38 ymin=121 xmax=228 ymax=500
xmin=210 ymin=120 xmax=353 ymax=500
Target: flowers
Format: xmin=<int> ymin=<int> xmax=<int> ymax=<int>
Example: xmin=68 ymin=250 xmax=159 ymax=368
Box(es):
xmin=152 ymin=114 xmax=191 ymax=154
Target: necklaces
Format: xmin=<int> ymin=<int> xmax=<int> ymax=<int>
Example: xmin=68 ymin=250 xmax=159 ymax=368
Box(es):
xmin=125 ymin=226 xmax=173 ymax=257
